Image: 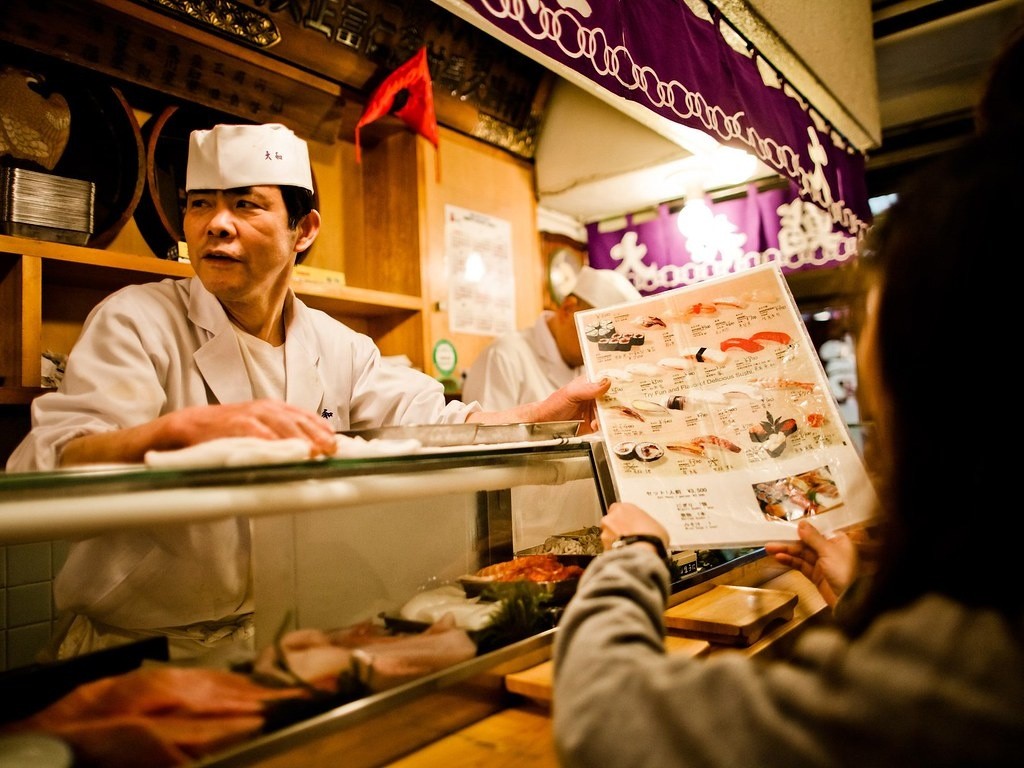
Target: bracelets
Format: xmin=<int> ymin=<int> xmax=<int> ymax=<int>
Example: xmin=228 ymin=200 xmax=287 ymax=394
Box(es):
xmin=612 ymin=534 xmax=671 ymax=564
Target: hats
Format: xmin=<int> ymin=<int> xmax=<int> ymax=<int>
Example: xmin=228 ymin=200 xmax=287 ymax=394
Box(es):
xmin=572 ymin=265 xmax=643 ymax=308
xmin=185 ymin=123 xmax=314 ymax=196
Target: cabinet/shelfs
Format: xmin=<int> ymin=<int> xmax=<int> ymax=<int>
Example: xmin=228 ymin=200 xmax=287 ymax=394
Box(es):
xmin=0 ymin=125 xmax=445 ymax=389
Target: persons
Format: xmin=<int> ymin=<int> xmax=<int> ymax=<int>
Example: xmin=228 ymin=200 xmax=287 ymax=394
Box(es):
xmin=463 ymin=265 xmax=645 ymax=413
xmin=6 ymin=122 xmax=612 ymax=671
xmin=554 ymin=30 xmax=1024 ymax=768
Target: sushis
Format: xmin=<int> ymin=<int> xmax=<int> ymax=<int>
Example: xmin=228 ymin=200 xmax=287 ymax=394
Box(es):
xmin=583 ymin=290 xmax=840 ymax=517
